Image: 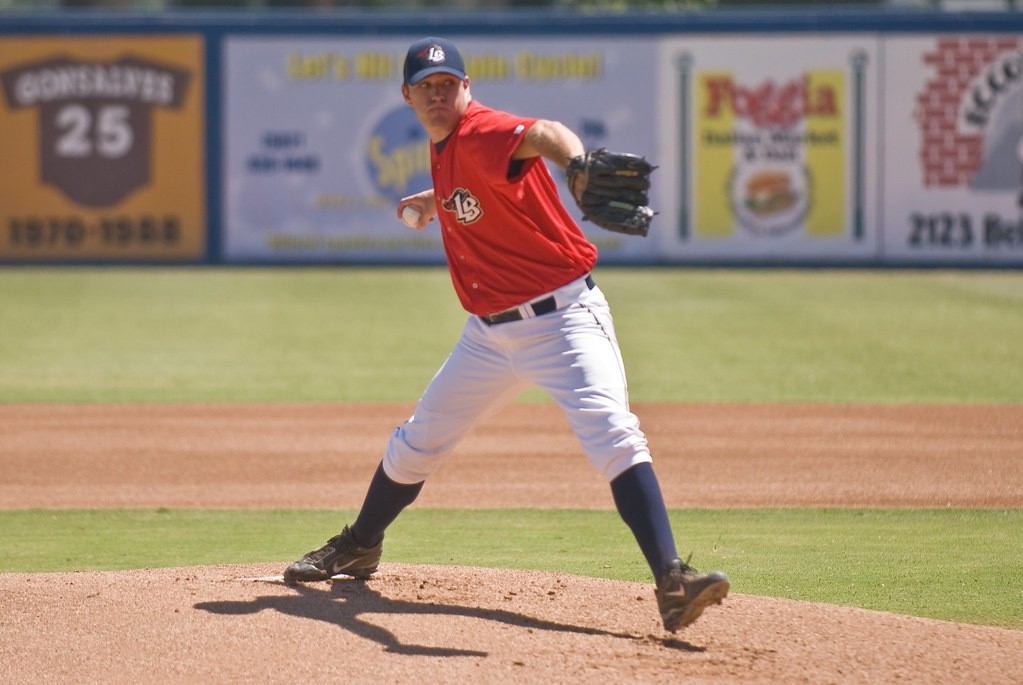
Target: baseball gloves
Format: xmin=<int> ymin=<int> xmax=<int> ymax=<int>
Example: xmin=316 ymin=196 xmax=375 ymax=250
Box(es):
xmin=565 ymin=148 xmax=654 ymax=237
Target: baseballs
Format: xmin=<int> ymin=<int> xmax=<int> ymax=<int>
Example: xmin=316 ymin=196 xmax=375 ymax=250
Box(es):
xmin=401 ymin=204 xmax=424 ymax=228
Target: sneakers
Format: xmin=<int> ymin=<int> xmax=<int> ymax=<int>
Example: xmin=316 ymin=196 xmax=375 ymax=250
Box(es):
xmin=653 ymin=551 xmax=730 ymax=634
xmin=283 ymin=523 xmax=385 ymax=581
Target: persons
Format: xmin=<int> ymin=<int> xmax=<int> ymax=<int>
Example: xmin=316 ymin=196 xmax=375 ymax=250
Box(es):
xmin=284 ymin=36 xmax=729 ymax=631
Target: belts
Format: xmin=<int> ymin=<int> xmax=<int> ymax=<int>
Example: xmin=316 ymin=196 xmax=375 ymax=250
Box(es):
xmin=480 ymin=274 xmax=596 ymax=324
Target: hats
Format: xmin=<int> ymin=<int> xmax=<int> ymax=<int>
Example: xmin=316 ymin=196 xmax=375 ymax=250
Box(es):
xmin=404 ymin=37 xmax=465 ymax=86
